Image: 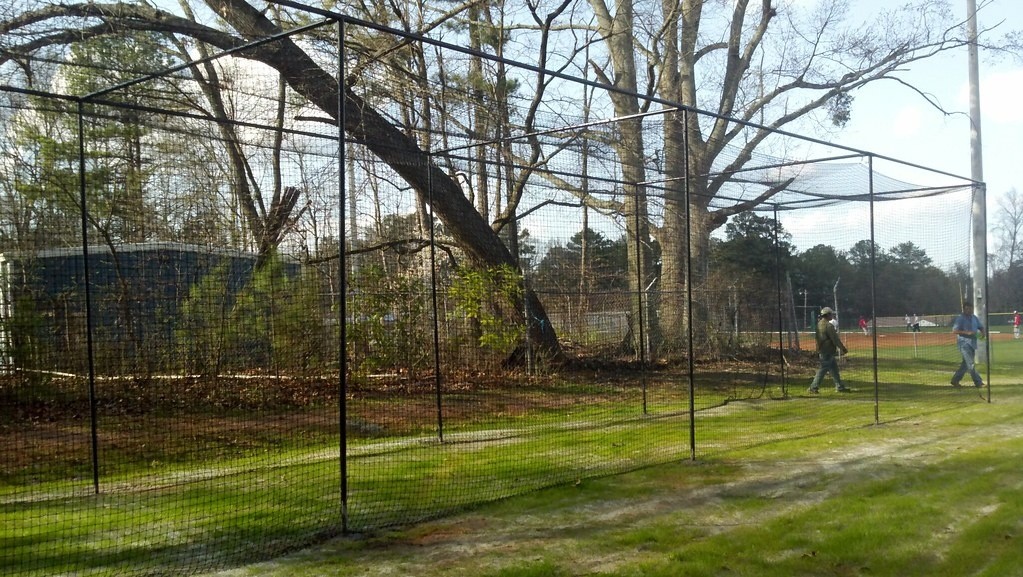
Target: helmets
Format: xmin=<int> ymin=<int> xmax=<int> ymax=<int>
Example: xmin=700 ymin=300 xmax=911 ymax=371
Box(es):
xmin=820 ymin=306 xmax=834 ymax=315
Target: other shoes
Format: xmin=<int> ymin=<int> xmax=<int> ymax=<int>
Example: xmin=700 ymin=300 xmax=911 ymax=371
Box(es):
xmin=951 ymin=381 xmax=962 ymax=387
xmin=837 ymin=388 xmax=848 ymax=392
xmin=807 ymin=388 xmax=819 ymax=394
xmin=976 ymin=383 xmax=987 ymax=388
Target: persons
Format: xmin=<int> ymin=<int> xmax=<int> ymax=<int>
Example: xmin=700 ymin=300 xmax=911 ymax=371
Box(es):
xmin=828 ymin=312 xmax=839 ymax=333
xmin=807 ymin=307 xmax=850 ymax=394
xmin=1013 ymin=310 xmax=1021 ymax=339
xmin=951 ymin=303 xmax=984 ymax=388
xmin=904 ymin=314 xmax=914 ymax=332
xmin=859 ymin=316 xmax=870 ymax=336
xmin=913 ymin=314 xmax=920 ymax=332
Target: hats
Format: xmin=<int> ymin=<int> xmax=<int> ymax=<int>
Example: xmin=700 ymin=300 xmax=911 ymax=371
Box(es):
xmin=1014 ymin=311 xmax=1018 ymax=314
